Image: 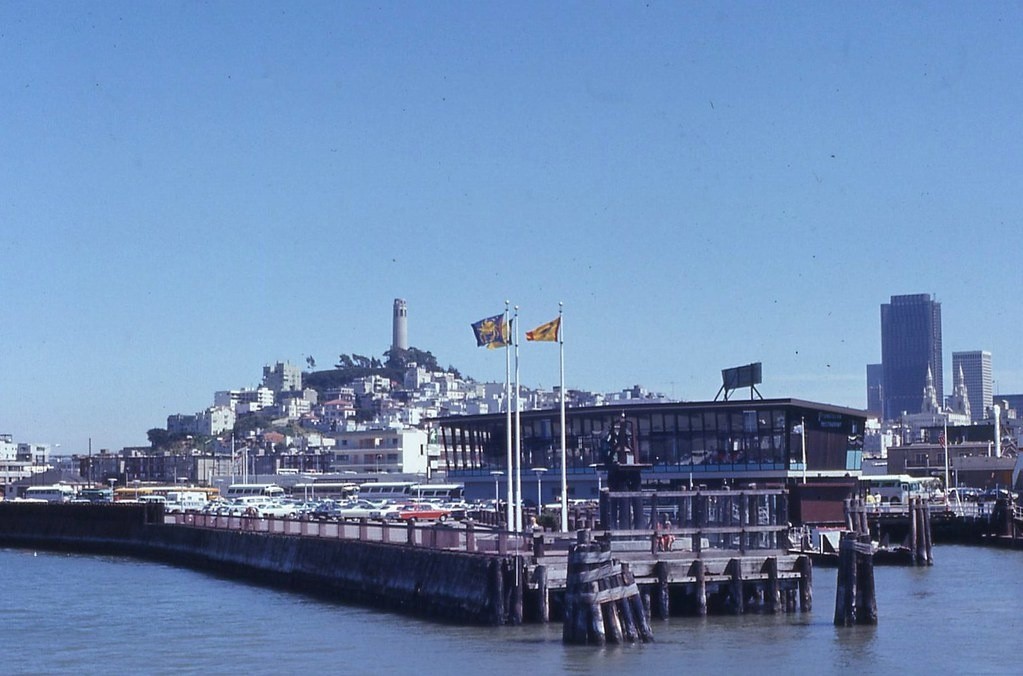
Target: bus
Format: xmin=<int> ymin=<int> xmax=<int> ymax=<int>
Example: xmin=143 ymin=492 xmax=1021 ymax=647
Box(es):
xmin=854 ymin=474 xmax=942 ymax=505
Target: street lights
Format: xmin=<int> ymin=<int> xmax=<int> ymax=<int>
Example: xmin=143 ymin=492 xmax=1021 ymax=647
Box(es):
xmin=416 ymin=474 xmax=424 ymax=498
xmin=308 ymin=477 xmax=318 ymax=501
xmin=301 ymin=475 xmax=310 ymax=501
xmin=216 ymin=479 xmax=224 ymax=495
xmin=108 ymin=479 xmax=118 ymax=501
xmin=133 ymin=479 xmax=140 ymax=500
xmin=793 ymin=416 xmax=806 ymax=484
xmin=60 ymin=481 xmax=66 ymax=502
xmin=531 ymin=468 xmax=548 ymax=518
xmin=489 ymin=471 xmax=504 ymax=512
xmin=177 ymin=477 xmax=188 ymax=511
xmin=589 ymin=463 xmax=605 ymax=500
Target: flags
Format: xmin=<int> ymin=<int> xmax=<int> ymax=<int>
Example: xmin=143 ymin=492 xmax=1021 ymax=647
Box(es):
xmin=471 ymin=313 xmax=513 ymax=350
xmin=938 ymin=426 xmax=945 ymax=448
xmin=793 ymin=425 xmax=802 ymax=435
xmin=525 ymin=316 xmax=560 ymax=342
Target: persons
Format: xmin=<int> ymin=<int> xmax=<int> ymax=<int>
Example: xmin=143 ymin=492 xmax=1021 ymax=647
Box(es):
xmin=655 ymin=521 xmax=676 ymax=552
xmin=524 ymin=516 xmax=539 ymax=550
xmin=845 ymin=491 xmax=881 ymax=517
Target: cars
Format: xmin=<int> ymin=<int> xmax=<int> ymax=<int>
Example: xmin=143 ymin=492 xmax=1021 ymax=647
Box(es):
xmin=25 ymin=480 xmax=598 ymax=524
xmin=947 ymin=487 xmax=1019 ymax=502
